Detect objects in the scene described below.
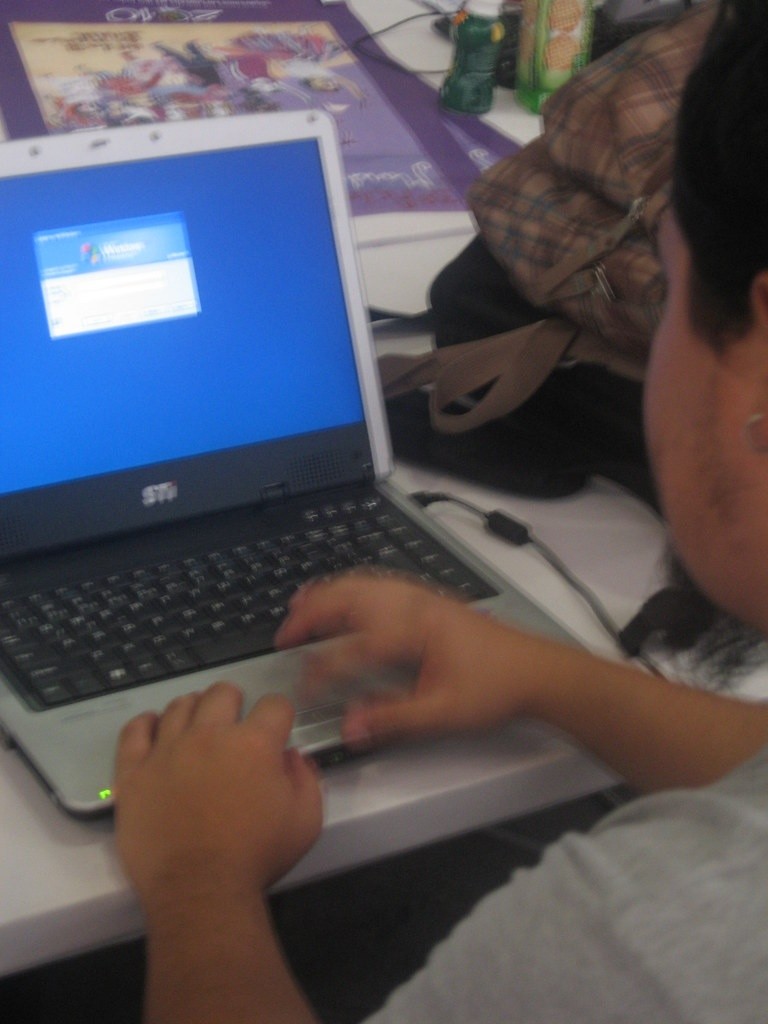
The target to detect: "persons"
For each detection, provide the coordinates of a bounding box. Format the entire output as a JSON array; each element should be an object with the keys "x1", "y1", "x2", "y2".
[
  {"x1": 378, "y1": 0, "x2": 726, "y2": 521},
  {"x1": 105, "y1": 0, "x2": 766, "y2": 1024}
]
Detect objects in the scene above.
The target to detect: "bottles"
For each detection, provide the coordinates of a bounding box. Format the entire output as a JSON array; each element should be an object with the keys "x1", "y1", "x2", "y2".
[
  {"x1": 441, "y1": 1, "x2": 506, "y2": 119},
  {"x1": 515, "y1": 0, "x2": 596, "y2": 114}
]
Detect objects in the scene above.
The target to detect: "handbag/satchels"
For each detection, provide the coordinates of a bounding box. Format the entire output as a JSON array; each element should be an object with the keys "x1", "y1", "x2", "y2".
[{"x1": 366, "y1": 0, "x2": 719, "y2": 519}]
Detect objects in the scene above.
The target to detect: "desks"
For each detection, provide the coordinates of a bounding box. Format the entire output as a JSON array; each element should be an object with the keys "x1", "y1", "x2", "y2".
[{"x1": 2, "y1": 0, "x2": 767, "y2": 982}]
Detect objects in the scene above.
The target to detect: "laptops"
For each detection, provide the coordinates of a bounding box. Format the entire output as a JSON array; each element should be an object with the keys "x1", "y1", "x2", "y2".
[{"x1": 1, "y1": 106, "x2": 594, "y2": 822}]
